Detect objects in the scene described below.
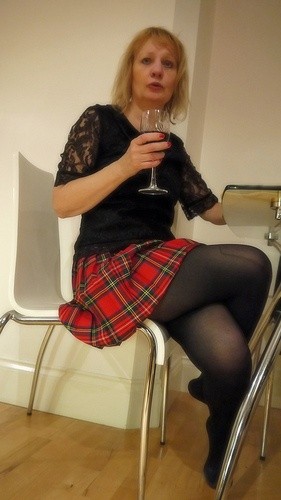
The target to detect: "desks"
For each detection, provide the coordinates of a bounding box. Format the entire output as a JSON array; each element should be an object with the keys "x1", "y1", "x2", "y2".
[{"x1": 212, "y1": 183, "x2": 281, "y2": 500}]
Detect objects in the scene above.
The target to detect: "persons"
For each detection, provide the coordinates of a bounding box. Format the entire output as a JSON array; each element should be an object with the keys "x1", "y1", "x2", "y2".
[{"x1": 51, "y1": 26, "x2": 272, "y2": 490}]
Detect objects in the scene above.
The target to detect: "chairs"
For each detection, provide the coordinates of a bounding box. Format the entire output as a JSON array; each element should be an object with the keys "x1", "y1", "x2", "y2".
[{"x1": 0, "y1": 152, "x2": 173, "y2": 499}]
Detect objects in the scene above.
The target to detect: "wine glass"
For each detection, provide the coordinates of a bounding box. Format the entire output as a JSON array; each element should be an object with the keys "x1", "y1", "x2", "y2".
[{"x1": 137, "y1": 109, "x2": 169, "y2": 195}]
